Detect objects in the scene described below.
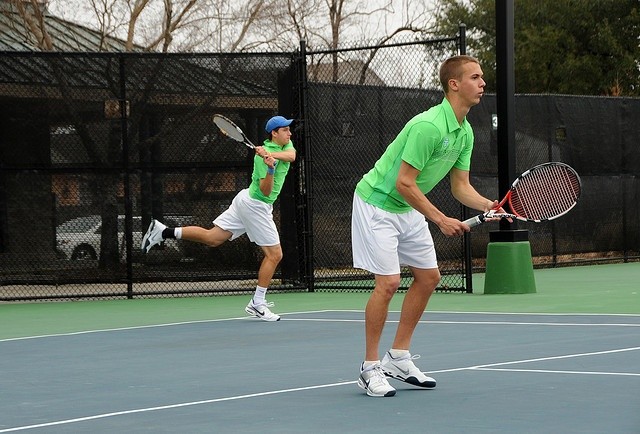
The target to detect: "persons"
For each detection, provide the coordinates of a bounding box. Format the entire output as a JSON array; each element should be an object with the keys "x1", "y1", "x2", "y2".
[
  {"x1": 351, "y1": 56, "x2": 514, "y2": 397},
  {"x1": 141, "y1": 116, "x2": 297, "y2": 322}
]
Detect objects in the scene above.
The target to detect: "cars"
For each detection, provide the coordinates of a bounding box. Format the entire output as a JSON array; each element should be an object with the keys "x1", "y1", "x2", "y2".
[
  {"x1": 158, "y1": 214, "x2": 203, "y2": 227},
  {"x1": 56, "y1": 215, "x2": 194, "y2": 267}
]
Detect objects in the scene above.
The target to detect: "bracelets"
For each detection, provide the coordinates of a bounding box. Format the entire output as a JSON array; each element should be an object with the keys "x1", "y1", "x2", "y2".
[{"x1": 267, "y1": 167, "x2": 275, "y2": 175}]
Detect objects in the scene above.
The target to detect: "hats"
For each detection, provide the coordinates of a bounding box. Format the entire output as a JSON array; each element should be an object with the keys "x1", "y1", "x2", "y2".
[{"x1": 265, "y1": 116, "x2": 295, "y2": 133}]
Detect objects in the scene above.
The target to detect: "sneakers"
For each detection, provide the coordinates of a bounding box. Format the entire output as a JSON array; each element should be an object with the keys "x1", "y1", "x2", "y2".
[
  {"x1": 245, "y1": 298, "x2": 281, "y2": 322},
  {"x1": 381, "y1": 351, "x2": 436, "y2": 389},
  {"x1": 357, "y1": 361, "x2": 396, "y2": 397},
  {"x1": 141, "y1": 220, "x2": 169, "y2": 254}
]
